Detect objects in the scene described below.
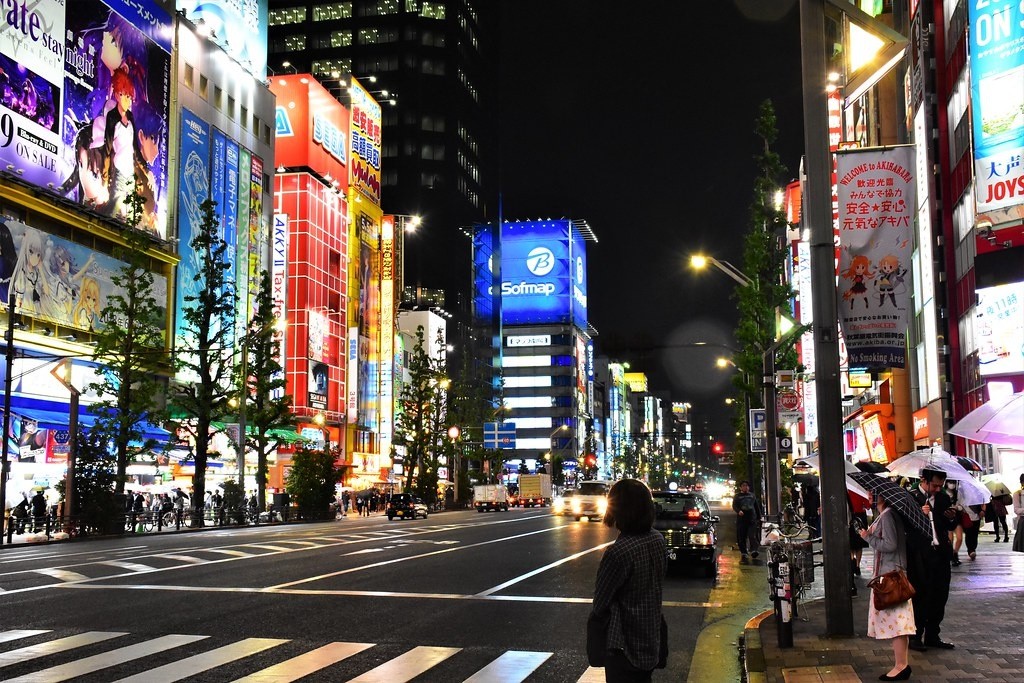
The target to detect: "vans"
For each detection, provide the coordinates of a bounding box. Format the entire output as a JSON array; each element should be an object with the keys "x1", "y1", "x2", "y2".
[{"x1": 574, "y1": 480, "x2": 617, "y2": 522}]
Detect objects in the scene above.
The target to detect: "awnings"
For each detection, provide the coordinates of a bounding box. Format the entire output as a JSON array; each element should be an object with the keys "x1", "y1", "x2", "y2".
[
  {"x1": 0, "y1": 406, "x2": 171, "y2": 442},
  {"x1": 150, "y1": 446, "x2": 225, "y2": 468}
]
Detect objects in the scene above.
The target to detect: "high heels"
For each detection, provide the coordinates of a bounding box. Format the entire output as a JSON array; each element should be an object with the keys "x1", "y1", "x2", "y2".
[{"x1": 878, "y1": 665, "x2": 911, "y2": 681}]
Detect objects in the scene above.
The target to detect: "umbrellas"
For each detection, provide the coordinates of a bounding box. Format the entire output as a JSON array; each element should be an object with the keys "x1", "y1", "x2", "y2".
[
  {"x1": 340, "y1": 486, "x2": 379, "y2": 498},
  {"x1": 803, "y1": 447, "x2": 1012, "y2": 541},
  {"x1": 947, "y1": 393, "x2": 1024, "y2": 446},
  {"x1": 792, "y1": 474, "x2": 818, "y2": 482},
  {"x1": 5, "y1": 484, "x2": 51, "y2": 508},
  {"x1": 124, "y1": 481, "x2": 189, "y2": 499},
  {"x1": 1012, "y1": 474, "x2": 1024, "y2": 553}
]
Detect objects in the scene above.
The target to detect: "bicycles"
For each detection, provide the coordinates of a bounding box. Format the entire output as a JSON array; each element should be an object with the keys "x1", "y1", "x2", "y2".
[
  {"x1": 762, "y1": 522, "x2": 817, "y2": 622},
  {"x1": 162, "y1": 509, "x2": 201, "y2": 528},
  {"x1": 124, "y1": 507, "x2": 155, "y2": 531}
]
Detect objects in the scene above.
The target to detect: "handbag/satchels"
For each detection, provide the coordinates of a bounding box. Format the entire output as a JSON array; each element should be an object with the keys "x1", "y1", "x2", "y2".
[
  {"x1": 946, "y1": 517, "x2": 957, "y2": 531},
  {"x1": 963, "y1": 512, "x2": 973, "y2": 528},
  {"x1": 586, "y1": 608, "x2": 670, "y2": 670},
  {"x1": 867, "y1": 568, "x2": 916, "y2": 610}
]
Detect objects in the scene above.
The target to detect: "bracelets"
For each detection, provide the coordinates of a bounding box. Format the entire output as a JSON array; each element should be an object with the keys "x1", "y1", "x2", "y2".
[{"x1": 981, "y1": 510, "x2": 985, "y2": 512}]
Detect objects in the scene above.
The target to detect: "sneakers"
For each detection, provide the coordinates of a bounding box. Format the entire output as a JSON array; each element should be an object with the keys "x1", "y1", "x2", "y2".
[{"x1": 741, "y1": 555, "x2": 749, "y2": 563}]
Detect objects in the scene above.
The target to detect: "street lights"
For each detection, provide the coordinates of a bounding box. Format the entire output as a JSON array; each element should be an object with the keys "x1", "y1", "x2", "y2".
[
  {"x1": 716, "y1": 355, "x2": 756, "y2": 495},
  {"x1": 550, "y1": 424, "x2": 568, "y2": 480},
  {"x1": 688, "y1": 253, "x2": 781, "y2": 525}
]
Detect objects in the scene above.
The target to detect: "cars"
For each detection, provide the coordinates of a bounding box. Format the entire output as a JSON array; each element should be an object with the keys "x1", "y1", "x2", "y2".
[
  {"x1": 511, "y1": 491, "x2": 518, "y2": 508},
  {"x1": 560, "y1": 489, "x2": 575, "y2": 516},
  {"x1": 386, "y1": 494, "x2": 429, "y2": 521},
  {"x1": 652, "y1": 490, "x2": 720, "y2": 579}
]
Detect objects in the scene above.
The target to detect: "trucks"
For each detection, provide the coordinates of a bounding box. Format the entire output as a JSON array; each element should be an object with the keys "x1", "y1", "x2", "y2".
[
  {"x1": 518, "y1": 473, "x2": 553, "y2": 508},
  {"x1": 473, "y1": 484, "x2": 509, "y2": 513}
]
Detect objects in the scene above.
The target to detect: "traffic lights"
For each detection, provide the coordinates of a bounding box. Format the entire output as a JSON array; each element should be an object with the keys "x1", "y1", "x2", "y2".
[
  {"x1": 588, "y1": 455, "x2": 596, "y2": 466},
  {"x1": 713, "y1": 443, "x2": 722, "y2": 452}
]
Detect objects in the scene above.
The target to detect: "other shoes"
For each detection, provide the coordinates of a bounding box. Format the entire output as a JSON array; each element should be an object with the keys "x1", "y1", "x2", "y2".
[
  {"x1": 969, "y1": 552, "x2": 976, "y2": 561},
  {"x1": 952, "y1": 560, "x2": 961, "y2": 567},
  {"x1": 995, "y1": 537, "x2": 1000, "y2": 542},
  {"x1": 751, "y1": 551, "x2": 759, "y2": 558},
  {"x1": 909, "y1": 640, "x2": 928, "y2": 652},
  {"x1": 1004, "y1": 537, "x2": 1009, "y2": 542},
  {"x1": 924, "y1": 640, "x2": 955, "y2": 649}
]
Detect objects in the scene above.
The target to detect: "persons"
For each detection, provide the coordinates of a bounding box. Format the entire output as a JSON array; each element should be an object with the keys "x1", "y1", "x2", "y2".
[
  {"x1": 991, "y1": 495, "x2": 1009, "y2": 543},
  {"x1": 250, "y1": 489, "x2": 258, "y2": 506},
  {"x1": 944, "y1": 479, "x2": 963, "y2": 566},
  {"x1": 908, "y1": 469, "x2": 955, "y2": 652},
  {"x1": 733, "y1": 480, "x2": 762, "y2": 563},
  {"x1": 802, "y1": 480, "x2": 822, "y2": 543},
  {"x1": 12, "y1": 491, "x2": 47, "y2": 535},
  {"x1": 858, "y1": 496, "x2": 917, "y2": 681},
  {"x1": 342, "y1": 491, "x2": 380, "y2": 516},
  {"x1": 586, "y1": 479, "x2": 668, "y2": 683},
  {"x1": 848, "y1": 490, "x2": 873, "y2": 575},
  {"x1": 204, "y1": 489, "x2": 222, "y2": 523},
  {"x1": 962, "y1": 504, "x2": 986, "y2": 559},
  {"x1": 125, "y1": 490, "x2": 184, "y2": 527}
]
